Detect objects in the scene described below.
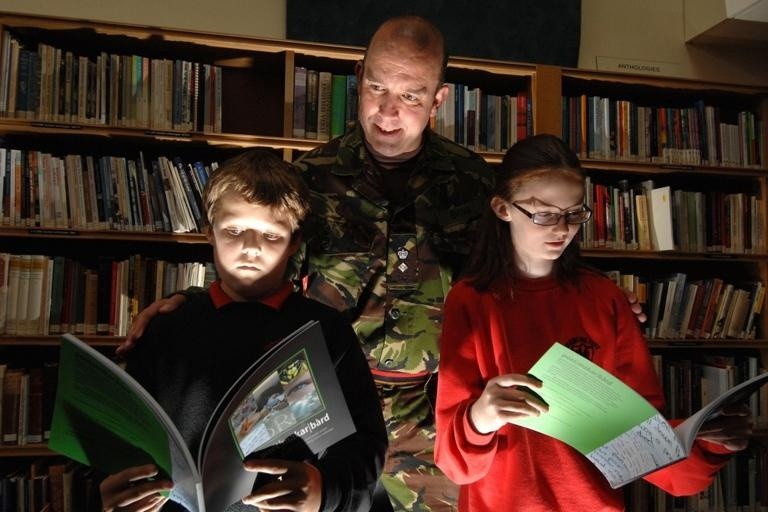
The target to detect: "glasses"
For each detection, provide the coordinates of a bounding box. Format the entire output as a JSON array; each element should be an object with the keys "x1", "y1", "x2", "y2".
[{"x1": 511, "y1": 202, "x2": 592, "y2": 226}]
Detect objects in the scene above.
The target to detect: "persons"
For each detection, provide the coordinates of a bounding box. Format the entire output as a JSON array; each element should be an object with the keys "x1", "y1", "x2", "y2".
[
  {"x1": 68, "y1": 146, "x2": 389, "y2": 512},
  {"x1": 116, "y1": 15, "x2": 647, "y2": 512},
  {"x1": 433, "y1": 136, "x2": 752, "y2": 512}
]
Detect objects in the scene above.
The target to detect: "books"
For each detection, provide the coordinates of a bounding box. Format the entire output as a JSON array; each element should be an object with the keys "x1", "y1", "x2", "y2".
[
  {"x1": 623, "y1": 442, "x2": 768, "y2": 512},
  {"x1": 294, "y1": 66, "x2": 358, "y2": 143},
  {"x1": 48, "y1": 320, "x2": 356, "y2": 512},
  {"x1": 0, "y1": 146, "x2": 219, "y2": 236},
  {"x1": 0, "y1": 31, "x2": 223, "y2": 135},
  {"x1": 429, "y1": 82, "x2": 533, "y2": 152},
  {"x1": 562, "y1": 94, "x2": 765, "y2": 170},
  {"x1": 1, "y1": 364, "x2": 101, "y2": 512},
  {"x1": 579, "y1": 178, "x2": 767, "y2": 256},
  {"x1": 606, "y1": 270, "x2": 766, "y2": 340},
  {"x1": 504, "y1": 342, "x2": 768, "y2": 488},
  {"x1": 0, "y1": 252, "x2": 220, "y2": 339},
  {"x1": 647, "y1": 353, "x2": 768, "y2": 426}
]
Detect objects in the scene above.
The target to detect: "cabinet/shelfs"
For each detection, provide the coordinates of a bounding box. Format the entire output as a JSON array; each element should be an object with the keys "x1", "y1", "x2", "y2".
[{"x1": 0, "y1": 10, "x2": 768, "y2": 512}]
